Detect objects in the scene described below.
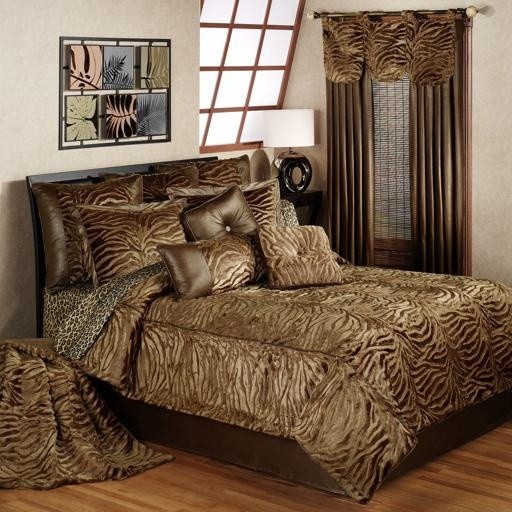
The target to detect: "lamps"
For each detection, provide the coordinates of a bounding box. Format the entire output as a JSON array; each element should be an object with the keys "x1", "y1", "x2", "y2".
[{"x1": 257, "y1": 107, "x2": 316, "y2": 196}]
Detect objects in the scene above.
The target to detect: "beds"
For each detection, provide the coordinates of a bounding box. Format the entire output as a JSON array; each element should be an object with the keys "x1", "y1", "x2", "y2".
[{"x1": 27, "y1": 156, "x2": 512, "y2": 504}]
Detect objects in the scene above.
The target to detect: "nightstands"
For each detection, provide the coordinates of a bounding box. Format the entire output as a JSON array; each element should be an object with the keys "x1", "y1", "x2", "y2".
[{"x1": 280, "y1": 190, "x2": 323, "y2": 225}]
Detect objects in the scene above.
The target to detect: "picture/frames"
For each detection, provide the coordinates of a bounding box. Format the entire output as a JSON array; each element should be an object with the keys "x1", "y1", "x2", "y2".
[{"x1": 56, "y1": 35, "x2": 172, "y2": 152}]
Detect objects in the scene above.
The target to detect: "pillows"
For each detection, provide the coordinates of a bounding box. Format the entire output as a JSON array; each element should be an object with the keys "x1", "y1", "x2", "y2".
[{"x1": 31, "y1": 155, "x2": 343, "y2": 300}]
